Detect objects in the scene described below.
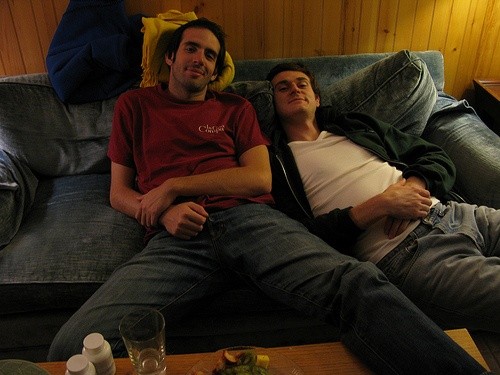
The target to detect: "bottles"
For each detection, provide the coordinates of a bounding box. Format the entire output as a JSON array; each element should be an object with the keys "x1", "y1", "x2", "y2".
[
  {"x1": 82, "y1": 333, "x2": 116, "y2": 375},
  {"x1": 64, "y1": 354, "x2": 96, "y2": 375}
]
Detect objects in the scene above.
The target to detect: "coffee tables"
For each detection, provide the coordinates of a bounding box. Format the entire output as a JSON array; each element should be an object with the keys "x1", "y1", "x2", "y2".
[{"x1": 37, "y1": 328, "x2": 490, "y2": 375}]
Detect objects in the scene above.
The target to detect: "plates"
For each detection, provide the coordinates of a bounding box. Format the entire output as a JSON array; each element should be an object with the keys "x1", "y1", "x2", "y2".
[{"x1": 185, "y1": 346, "x2": 307, "y2": 375}]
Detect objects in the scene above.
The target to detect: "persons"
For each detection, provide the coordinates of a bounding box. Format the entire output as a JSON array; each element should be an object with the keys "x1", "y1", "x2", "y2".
[
  {"x1": 266, "y1": 62, "x2": 500, "y2": 322},
  {"x1": 46, "y1": 19, "x2": 413, "y2": 375}
]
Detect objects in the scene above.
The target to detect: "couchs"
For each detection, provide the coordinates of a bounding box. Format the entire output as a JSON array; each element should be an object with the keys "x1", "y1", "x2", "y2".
[{"x1": 0, "y1": 51, "x2": 500, "y2": 363}]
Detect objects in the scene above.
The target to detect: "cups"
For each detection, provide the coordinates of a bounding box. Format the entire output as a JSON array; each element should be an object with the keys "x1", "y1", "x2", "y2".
[{"x1": 119, "y1": 308, "x2": 167, "y2": 375}]
